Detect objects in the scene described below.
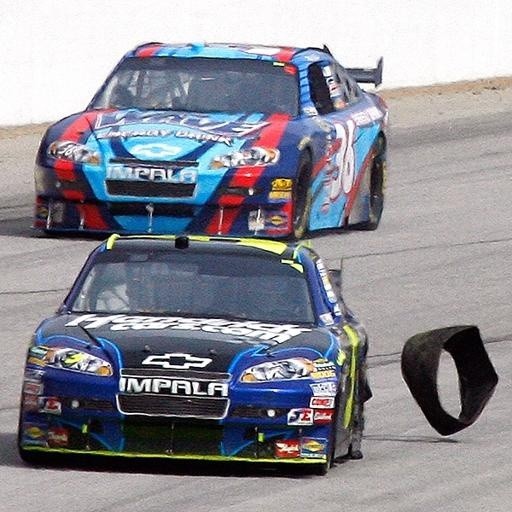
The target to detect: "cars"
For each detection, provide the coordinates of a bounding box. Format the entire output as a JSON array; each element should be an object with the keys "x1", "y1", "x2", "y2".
[
  {"x1": 17, "y1": 234, "x2": 371, "y2": 474},
  {"x1": 29, "y1": 40, "x2": 391, "y2": 239}
]
